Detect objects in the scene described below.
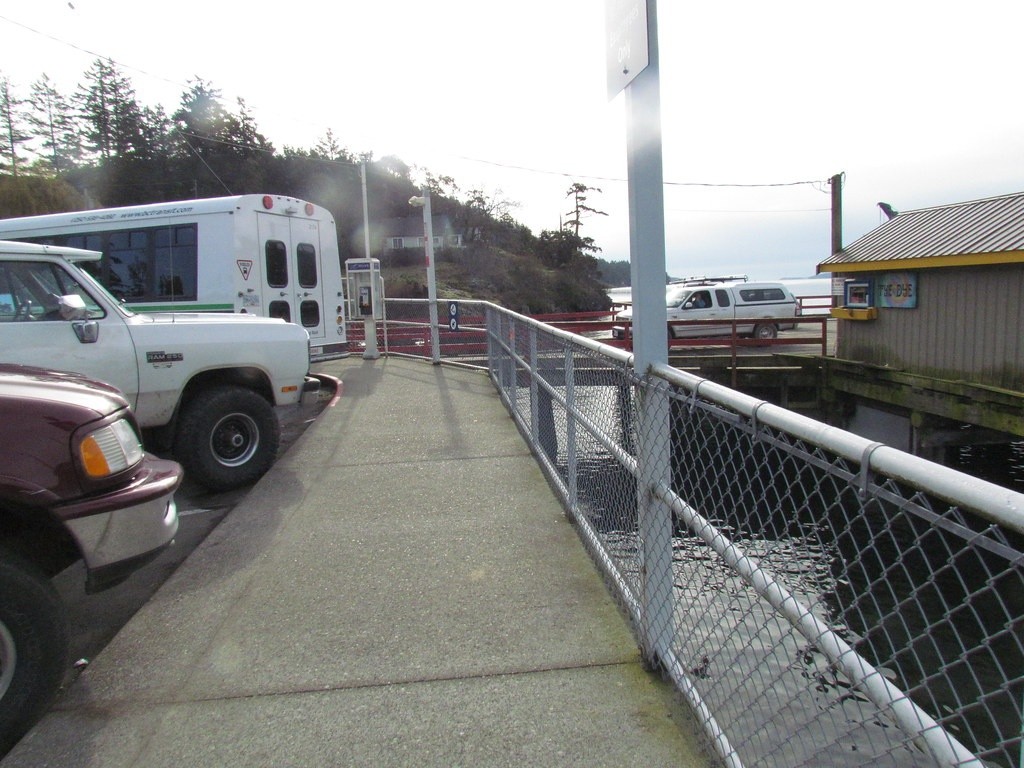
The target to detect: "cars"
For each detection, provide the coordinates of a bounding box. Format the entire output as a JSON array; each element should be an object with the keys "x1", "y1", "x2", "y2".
[{"x1": 0, "y1": 361, "x2": 184, "y2": 730}]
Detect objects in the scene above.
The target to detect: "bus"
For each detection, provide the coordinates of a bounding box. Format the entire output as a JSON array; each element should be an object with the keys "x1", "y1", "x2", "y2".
[{"x1": 0, "y1": 191, "x2": 352, "y2": 364}]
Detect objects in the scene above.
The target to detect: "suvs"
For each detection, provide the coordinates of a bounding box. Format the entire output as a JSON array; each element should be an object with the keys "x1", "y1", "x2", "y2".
[{"x1": 1, "y1": 239, "x2": 323, "y2": 495}]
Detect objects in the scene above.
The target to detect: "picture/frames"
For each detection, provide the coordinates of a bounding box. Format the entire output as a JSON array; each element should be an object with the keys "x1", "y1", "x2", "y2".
[{"x1": 843, "y1": 278, "x2": 874, "y2": 309}]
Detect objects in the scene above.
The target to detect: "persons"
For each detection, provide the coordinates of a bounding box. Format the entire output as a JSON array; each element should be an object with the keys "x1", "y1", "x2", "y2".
[{"x1": 692, "y1": 294, "x2": 706, "y2": 307}]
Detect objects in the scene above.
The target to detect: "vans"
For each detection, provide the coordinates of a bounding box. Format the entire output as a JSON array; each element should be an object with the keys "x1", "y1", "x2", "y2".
[{"x1": 612, "y1": 275, "x2": 803, "y2": 345}]
